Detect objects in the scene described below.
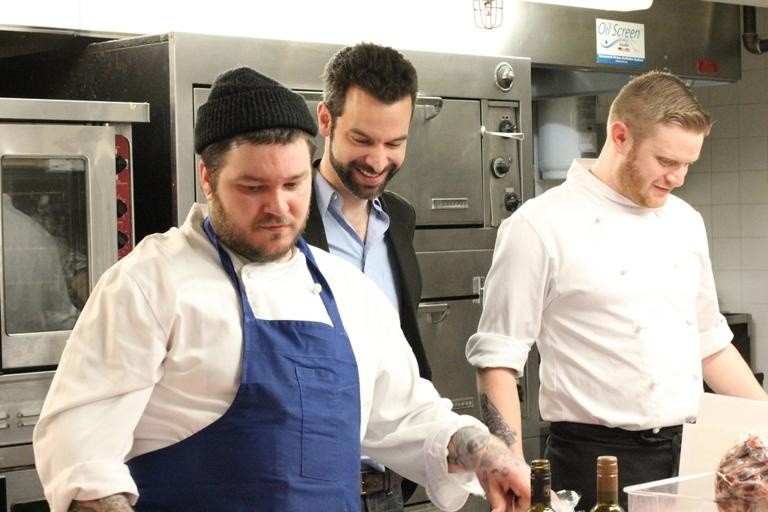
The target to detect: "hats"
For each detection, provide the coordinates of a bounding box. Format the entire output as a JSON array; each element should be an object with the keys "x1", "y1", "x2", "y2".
[{"x1": 195, "y1": 67, "x2": 317, "y2": 153}]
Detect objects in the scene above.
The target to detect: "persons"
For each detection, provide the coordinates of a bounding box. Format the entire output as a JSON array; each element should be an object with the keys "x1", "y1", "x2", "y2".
[
  {"x1": 466, "y1": 67, "x2": 768, "y2": 512},
  {"x1": 3, "y1": 168, "x2": 82, "y2": 334},
  {"x1": 302, "y1": 43, "x2": 434, "y2": 512}
]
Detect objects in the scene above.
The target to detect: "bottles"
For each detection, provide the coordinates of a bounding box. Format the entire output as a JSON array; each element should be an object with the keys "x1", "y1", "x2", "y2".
[
  {"x1": 589, "y1": 454, "x2": 623, "y2": 512},
  {"x1": 524, "y1": 459, "x2": 556, "y2": 512}
]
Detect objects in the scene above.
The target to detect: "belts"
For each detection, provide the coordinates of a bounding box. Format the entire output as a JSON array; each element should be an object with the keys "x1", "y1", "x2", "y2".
[
  {"x1": 551, "y1": 421, "x2": 683, "y2": 443},
  {"x1": 360, "y1": 470, "x2": 404, "y2": 495}
]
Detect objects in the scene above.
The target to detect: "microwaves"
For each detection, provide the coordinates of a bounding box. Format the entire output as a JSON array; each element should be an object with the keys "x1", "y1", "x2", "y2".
[{"x1": 0, "y1": 92, "x2": 150, "y2": 366}]
